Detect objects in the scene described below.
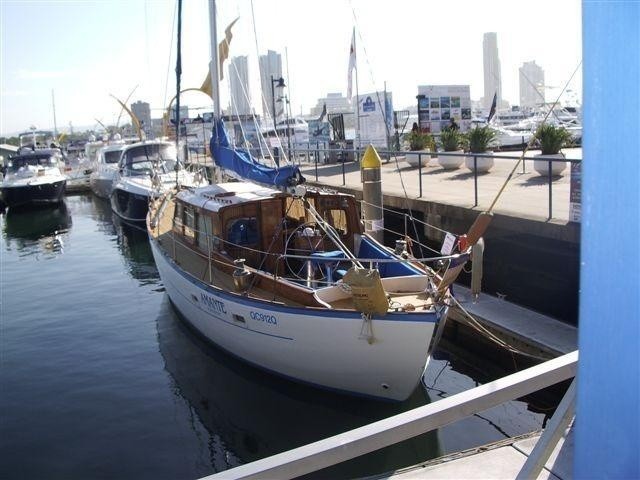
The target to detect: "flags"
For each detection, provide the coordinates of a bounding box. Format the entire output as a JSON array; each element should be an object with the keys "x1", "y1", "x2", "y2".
[
  {"x1": 488, "y1": 92, "x2": 498, "y2": 124},
  {"x1": 346, "y1": 27, "x2": 357, "y2": 101}
]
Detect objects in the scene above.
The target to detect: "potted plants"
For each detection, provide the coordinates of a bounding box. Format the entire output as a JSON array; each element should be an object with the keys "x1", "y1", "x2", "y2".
[
  {"x1": 534, "y1": 124, "x2": 571, "y2": 176},
  {"x1": 438, "y1": 126, "x2": 464, "y2": 170},
  {"x1": 463, "y1": 124, "x2": 501, "y2": 172},
  {"x1": 405, "y1": 130, "x2": 434, "y2": 168}
]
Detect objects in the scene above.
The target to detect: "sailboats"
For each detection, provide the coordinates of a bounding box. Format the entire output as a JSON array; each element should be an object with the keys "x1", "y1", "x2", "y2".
[
  {"x1": 153, "y1": 292, "x2": 445, "y2": 480},
  {"x1": 145, "y1": 1, "x2": 474, "y2": 415}
]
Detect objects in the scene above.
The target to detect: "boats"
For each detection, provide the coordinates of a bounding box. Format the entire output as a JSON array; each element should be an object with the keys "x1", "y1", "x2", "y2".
[
  {"x1": 112, "y1": 215, "x2": 161, "y2": 288},
  {"x1": 110, "y1": 138, "x2": 208, "y2": 234},
  {"x1": 4, "y1": 201, "x2": 72, "y2": 258},
  {"x1": 0, "y1": 87, "x2": 145, "y2": 210},
  {"x1": 472, "y1": 104, "x2": 581, "y2": 153},
  {"x1": 261, "y1": 117, "x2": 329, "y2": 150}
]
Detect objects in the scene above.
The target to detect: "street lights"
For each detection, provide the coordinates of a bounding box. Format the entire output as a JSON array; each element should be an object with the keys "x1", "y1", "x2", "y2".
[
  {"x1": 275, "y1": 93, "x2": 291, "y2": 164},
  {"x1": 269, "y1": 73, "x2": 286, "y2": 168}
]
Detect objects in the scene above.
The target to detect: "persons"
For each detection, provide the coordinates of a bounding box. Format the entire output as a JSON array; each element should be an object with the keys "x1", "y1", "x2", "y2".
[
  {"x1": 113, "y1": 131, "x2": 122, "y2": 140},
  {"x1": 44, "y1": 230, "x2": 64, "y2": 256},
  {"x1": 412, "y1": 122, "x2": 419, "y2": 137},
  {"x1": 87, "y1": 132, "x2": 96, "y2": 142},
  {"x1": 448, "y1": 117, "x2": 458, "y2": 131}
]
